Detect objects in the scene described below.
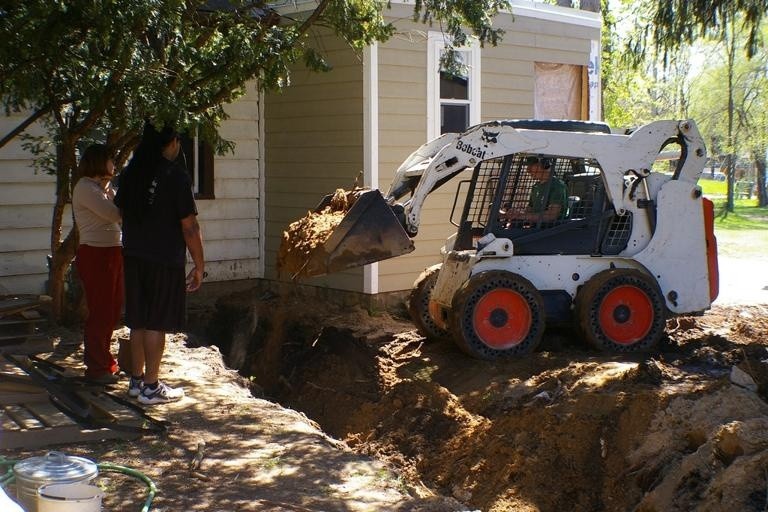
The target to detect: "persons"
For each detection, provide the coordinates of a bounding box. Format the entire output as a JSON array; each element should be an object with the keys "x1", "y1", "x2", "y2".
[
  {"x1": 113, "y1": 124, "x2": 206, "y2": 405},
  {"x1": 71, "y1": 143, "x2": 126, "y2": 383},
  {"x1": 499, "y1": 158, "x2": 576, "y2": 229}
]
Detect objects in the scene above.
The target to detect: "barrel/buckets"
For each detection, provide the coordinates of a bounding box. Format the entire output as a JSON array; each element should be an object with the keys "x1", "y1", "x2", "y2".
[
  {"x1": 12, "y1": 449, "x2": 99, "y2": 511},
  {"x1": 35, "y1": 483, "x2": 104, "y2": 511}
]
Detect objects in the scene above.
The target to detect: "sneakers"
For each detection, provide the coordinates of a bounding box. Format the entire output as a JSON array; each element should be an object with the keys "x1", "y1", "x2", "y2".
[
  {"x1": 138, "y1": 383, "x2": 183, "y2": 404},
  {"x1": 129, "y1": 379, "x2": 143, "y2": 395},
  {"x1": 87, "y1": 370, "x2": 127, "y2": 383}
]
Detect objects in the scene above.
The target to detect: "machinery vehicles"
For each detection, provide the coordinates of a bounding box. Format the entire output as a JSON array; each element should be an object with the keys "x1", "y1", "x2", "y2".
[{"x1": 281, "y1": 117, "x2": 722, "y2": 363}]
